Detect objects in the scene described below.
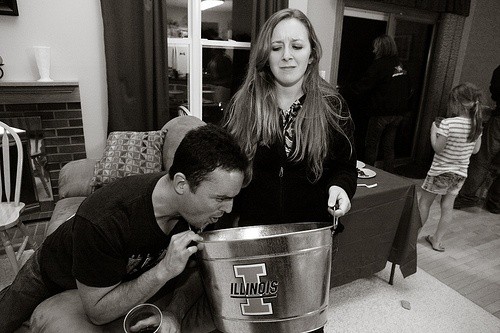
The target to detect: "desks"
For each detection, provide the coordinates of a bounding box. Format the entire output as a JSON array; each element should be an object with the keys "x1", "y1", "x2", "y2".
[{"x1": 332, "y1": 160, "x2": 423, "y2": 289}]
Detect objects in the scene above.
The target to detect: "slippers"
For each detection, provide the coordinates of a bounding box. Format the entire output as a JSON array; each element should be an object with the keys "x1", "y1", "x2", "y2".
[{"x1": 425, "y1": 235, "x2": 445, "y2": 251}]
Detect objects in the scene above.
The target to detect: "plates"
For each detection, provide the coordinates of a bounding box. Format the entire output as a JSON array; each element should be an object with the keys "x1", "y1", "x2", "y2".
[
  {"x1": 357, "y1": 169, "x2": 376, "y2": 178},
  {"x1": 357, "y1": 160, "x2": 365, "y2": 168}
]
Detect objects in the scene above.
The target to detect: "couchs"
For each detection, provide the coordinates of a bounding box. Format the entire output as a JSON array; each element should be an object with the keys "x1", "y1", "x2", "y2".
[{"x1": 29, "y1": 116, "x2": 207, "y2": 333}]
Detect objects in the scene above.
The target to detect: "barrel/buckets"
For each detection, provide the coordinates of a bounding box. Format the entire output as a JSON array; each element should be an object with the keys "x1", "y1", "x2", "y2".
[{"x1": 189, "y1": 206, "x2": 338, "y2": 333}]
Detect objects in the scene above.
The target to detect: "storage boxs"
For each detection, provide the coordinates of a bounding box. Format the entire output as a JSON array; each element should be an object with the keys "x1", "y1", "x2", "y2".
[{"x1": 202, "y1": 207, "x2": 338, "y2": 333}]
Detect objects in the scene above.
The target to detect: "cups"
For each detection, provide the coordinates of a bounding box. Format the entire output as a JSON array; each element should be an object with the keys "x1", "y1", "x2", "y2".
[
  {"x1": 123, "y1": 304, "x2": 178, "y2": 333},
  {"x1": 435, "y1": 117, "x2": 445, "y2": 136}
]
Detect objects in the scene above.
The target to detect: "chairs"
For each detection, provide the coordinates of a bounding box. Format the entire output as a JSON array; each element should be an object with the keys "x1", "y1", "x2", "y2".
[{"x1": 0, "y1": 121, "x2": 37, "y2": 277}]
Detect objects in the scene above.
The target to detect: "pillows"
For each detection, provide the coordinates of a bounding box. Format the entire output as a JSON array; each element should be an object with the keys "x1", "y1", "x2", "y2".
[{"x1": 91, "y1": 129, "x2": 168, "y2": 184}]
